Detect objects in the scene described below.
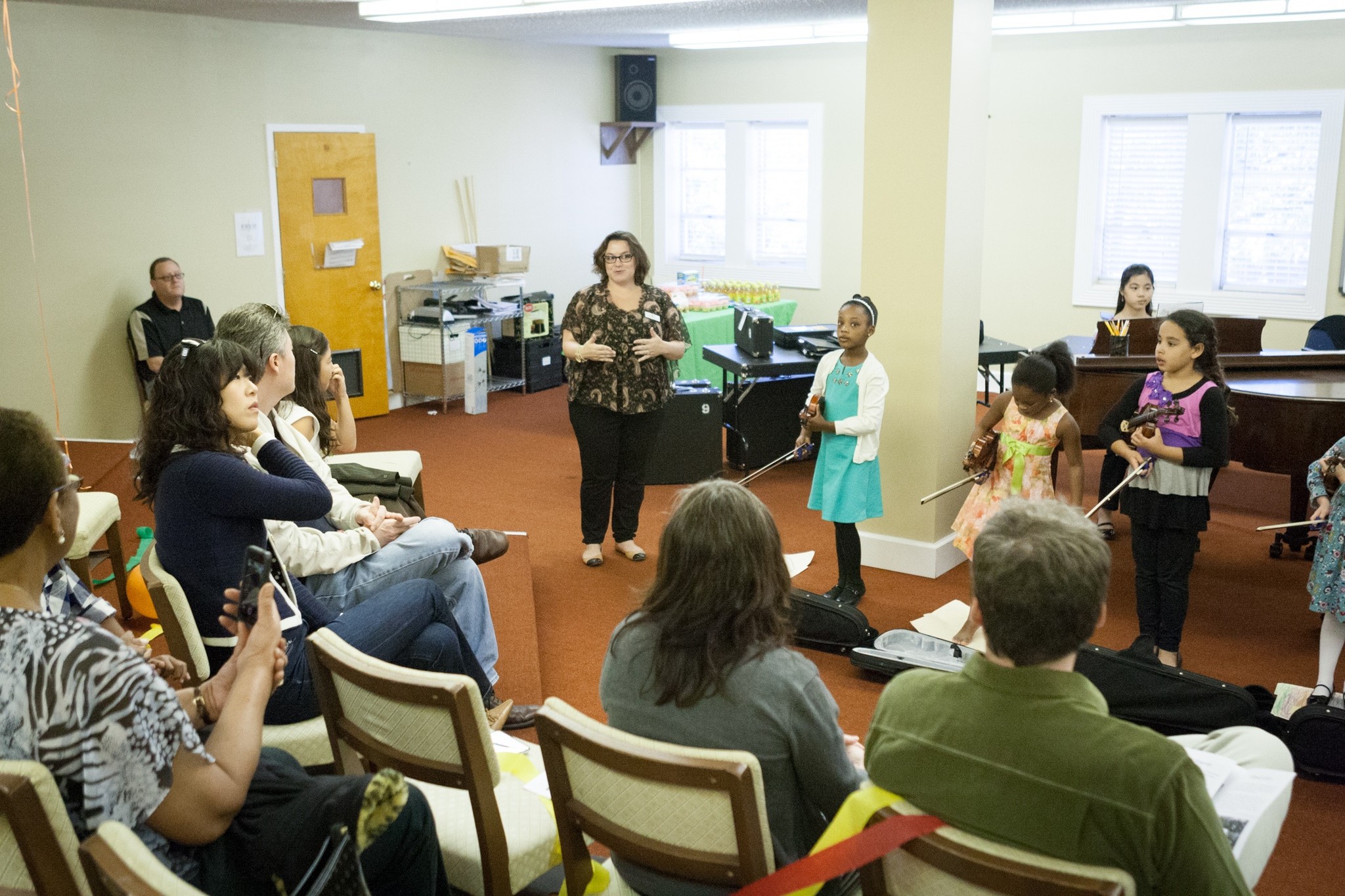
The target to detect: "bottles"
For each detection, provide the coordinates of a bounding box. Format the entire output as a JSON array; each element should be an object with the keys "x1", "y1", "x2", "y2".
[{"x1": 703, "y1": 279, "x2": 782, "y2": 304}]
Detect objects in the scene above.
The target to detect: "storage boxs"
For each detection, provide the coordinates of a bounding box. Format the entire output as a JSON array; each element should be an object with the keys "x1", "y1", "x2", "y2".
[
  {"x1": 734, "y1": 304, "x2": 774, "y2": 357},
  {"x1": 465, "y1": 327, "x2": 488, "y2": 414},
  {"x1": 398, "y1": 325, "x2": 470, "y2": 365},
  {"x1": 773, "y1": 323, "x2": 837, "y2": 351},
  {"x1": 641, "y1": 379, "x2": 724, "y2": 485},
  {"x1": 491, "y1": 337, "x2": 563, "y2": 392},
  {"x1": 501, "y1": 291, "x2": 553, "y2": 339},
  {"x1": 725, "y1": 373, "x2": 819, "y2": 470},
  {"x1": 477, "y1": 245, "x2": 530, "y2": 273}
]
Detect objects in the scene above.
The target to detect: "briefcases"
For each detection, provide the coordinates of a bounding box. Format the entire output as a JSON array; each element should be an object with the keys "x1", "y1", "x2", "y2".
[{"x1": 732, "y1": 305, "x2": 774, "y2": 358}]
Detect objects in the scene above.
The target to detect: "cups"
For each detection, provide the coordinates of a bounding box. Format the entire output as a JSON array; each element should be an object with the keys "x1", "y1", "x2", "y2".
[{"x1": 1109, "y1": 334, "x2": 1129, "y2": 356}]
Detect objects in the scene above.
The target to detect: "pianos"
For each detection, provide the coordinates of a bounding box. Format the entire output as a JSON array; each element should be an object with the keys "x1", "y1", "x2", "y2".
[{"x1": 1026, "y1": 304, "x2": 1345, "y2": 555}]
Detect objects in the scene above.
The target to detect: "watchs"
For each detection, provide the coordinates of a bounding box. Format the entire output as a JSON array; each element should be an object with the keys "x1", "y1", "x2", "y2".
[{"x1": 574, "y1": 346, "x2": 588, "y2": 364}]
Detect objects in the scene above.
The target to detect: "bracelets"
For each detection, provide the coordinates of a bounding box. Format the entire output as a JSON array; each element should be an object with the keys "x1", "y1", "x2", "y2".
[{"x1": 193, "y1": 686, "x2": 214, "y2": 725}]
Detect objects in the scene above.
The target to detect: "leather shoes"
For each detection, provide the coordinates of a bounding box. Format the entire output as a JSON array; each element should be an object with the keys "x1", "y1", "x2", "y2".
[
  {"x1": 1097, "y1": 522, "x2": 1116, "y2": 538},
  {"x1": 459, "y1": 527, "x2": 509, "y2": 564},
  {"x1": 823, "y1": 579, "x2": 866, "y2": 607},
  {"x1": 484, "y1": 696, "x2": 542, "y2": 731}
]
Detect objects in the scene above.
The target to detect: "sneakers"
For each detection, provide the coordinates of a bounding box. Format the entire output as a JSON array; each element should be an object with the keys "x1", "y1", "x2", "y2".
[{"x1": 1306, "y1": 684, "x2": 1335, "y2": 706}]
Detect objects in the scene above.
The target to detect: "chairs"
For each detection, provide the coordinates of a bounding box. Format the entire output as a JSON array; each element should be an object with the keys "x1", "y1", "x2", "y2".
[{"x1": 0, "y1": 451, "x2": 1135, "y2": 896}]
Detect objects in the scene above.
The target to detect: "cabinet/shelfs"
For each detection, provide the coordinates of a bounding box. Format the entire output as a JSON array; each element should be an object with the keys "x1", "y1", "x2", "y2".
[{"x1": 395, "y1": 277, "x2": 527, "y2": 414}]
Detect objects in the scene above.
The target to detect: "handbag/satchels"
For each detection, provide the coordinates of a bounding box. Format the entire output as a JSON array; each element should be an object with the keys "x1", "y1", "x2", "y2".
[
  {"x1": 272, "y1": 824, "x2": 374, "y2": 896},
  {"x1": 784, "y1": 587, "x2": 879, "y2": 657},
  {"x1": 1285, "y1": 704, "x2": 1344, "y2": 786},
  {"x1": 1075, "y1": 643, "x2": 1260, "y2": 740}
]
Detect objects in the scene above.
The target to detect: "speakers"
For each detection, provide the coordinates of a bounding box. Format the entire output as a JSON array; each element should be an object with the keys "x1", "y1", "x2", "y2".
[{"x1": 615, "y1": 54, "x2": 656, "y2": 122}]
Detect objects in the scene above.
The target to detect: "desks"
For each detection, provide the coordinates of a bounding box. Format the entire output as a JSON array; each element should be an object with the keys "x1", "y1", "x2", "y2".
[
  {"x1": 703, "y1": 332, "x2": 842, "y2": 408},
  {"x1": 668, "y1": 296, "x2": 797, "y2": 390},
  {"x1": 976, "y1": 335, "x2": 1029, "y2": 407}
]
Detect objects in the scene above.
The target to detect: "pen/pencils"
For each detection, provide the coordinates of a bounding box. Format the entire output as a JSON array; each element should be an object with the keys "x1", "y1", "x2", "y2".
[{"x1": 1104, "y1": 319, "x2": 1130, "y2": 336}]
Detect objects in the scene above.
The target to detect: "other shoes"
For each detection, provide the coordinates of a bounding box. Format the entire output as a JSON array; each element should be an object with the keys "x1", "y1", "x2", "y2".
[
  {"x1": 486, "y1": 699, "x2": 513, "y2": 732},
  {"x1": 581, "y1": 543, "x2": 604, "y2": 566},
  {"x1": 614, "y1": 540, "x2": 646, "y2": 560}
]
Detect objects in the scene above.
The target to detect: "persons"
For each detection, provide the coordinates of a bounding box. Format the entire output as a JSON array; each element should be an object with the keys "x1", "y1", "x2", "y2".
[
  {"x1": 1304, "y1": 435, "x2": 1345, "y2": 712},
  {"x1": 864, "y1": 500, "x2": 1297, "y2": 896},
  {"x1": 793, "y1": 294, "x2": 888, "y2": 606},
  {"x1": 559, "y1": 231, "x2": 692, "y2": 567},
  {"x1": 1081, "y1": 263, "x2": 1157, "y2": 540},
  {"x1": 599, "y1": 477, "x2": 883, "y2": 896},
  {"x1": 1094, "y1": 308, "x2": 1238, "y2": 670},
  {"x1": 0, "y1": 257, "x2": 548, "y2": 894},
  {"x1": 947, "y1": 338, "x2": 1085, "y2": 647}
]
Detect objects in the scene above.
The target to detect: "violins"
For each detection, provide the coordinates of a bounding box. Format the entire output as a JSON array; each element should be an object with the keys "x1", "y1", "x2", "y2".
[
  {"x1": 1120, "y1": 399, "x2": 1184, "y2": 446},
  {"x1": 1321, "y1": 451, "x2": 1345, "y2": 495},
  {"x1": 798, "y1": 394, "x2": 825, "y2": 428},
  {"x1": 962, "y1": 428, "x2": 1000, "y2": 472}
]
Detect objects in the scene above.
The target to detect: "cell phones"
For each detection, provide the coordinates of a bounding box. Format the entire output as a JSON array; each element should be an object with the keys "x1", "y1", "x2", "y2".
[{"x1": 236, "y1": 545, "x2": 271, "y2": 624}]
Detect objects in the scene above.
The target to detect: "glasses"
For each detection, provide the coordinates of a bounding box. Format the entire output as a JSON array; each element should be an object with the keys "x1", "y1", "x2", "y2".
[
  {"x1": 179, "y1": 337, "x2": 207, "y2": 370},
  {"x1": 48, "y1": 473, "x2": 84, "y2": 497},
  {"x1": 154, "y1": 273, "x2": 185, "y2": 282},
  {"x1": 603, "y1": 253, "x2": 633, "y2": 264}
]
